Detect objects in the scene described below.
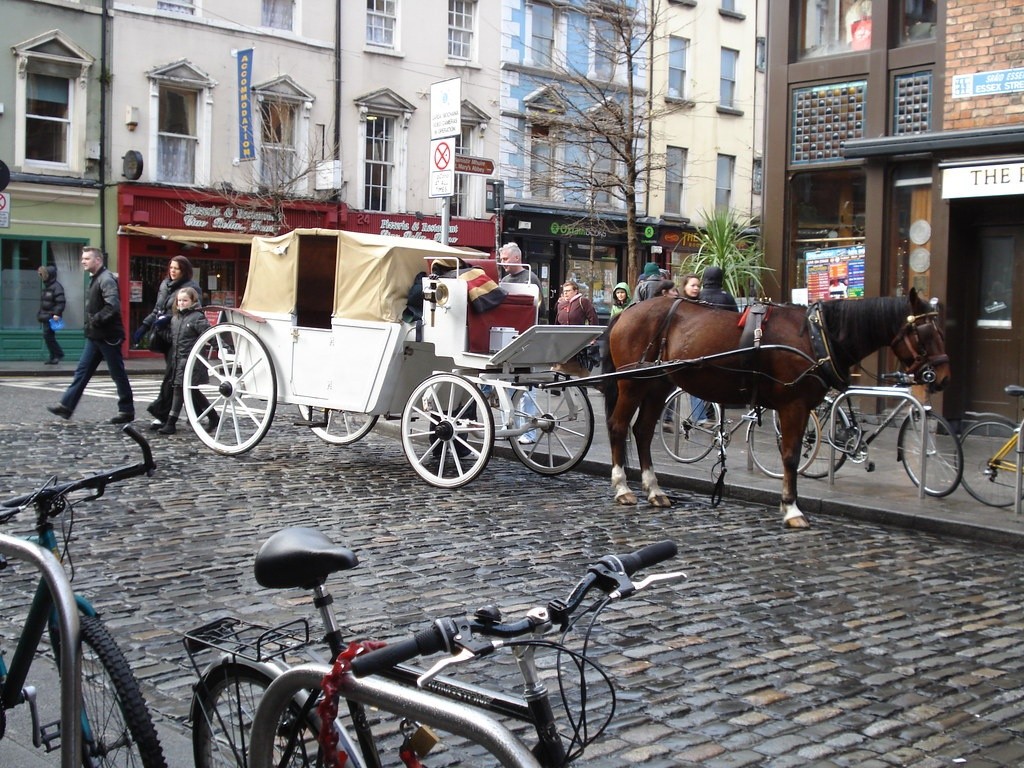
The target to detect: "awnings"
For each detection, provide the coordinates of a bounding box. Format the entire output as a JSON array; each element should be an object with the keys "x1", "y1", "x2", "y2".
[{"x1": 122, "y1": 225, "x2": 279, "y2": 246}]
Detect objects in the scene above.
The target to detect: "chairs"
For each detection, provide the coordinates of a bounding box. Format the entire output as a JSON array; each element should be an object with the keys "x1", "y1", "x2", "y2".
[{"x1": 426, "y1": 258, "x2": 540, "y2": 307}]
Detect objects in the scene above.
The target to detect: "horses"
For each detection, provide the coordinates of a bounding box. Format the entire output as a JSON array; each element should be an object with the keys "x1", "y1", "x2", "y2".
[{"x1": 601, "y1": 289, "x2": 950, "y2": 528}]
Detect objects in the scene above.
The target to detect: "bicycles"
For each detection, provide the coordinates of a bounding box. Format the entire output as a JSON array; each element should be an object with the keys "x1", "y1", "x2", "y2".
[
  {"x1": 660, "y1": 386, "x2": 821, "y2": 479},
  {"x1": 182, "y1": 524, "x2": 678, "y2": 768},
  {"x1": 775, "y1": 371, "x2": 965, "y2": 498},
  {"x1": 0, "y1": 424, "x2": 170, "y2": 768},
  {"x1": 953, "y1": 385, "x2": 1024, "y2": 508}
]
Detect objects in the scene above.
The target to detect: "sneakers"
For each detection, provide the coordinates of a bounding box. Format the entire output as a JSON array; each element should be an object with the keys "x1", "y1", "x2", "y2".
[
  {"x1": 459, "y1": 451, "x2": 480, "y2": 459},
  {"x1": 149, "y1": 418, "x2": 165, "y2": 431},
  {"x1": 433, "y1": 448, "x2": 453, "y2": 457},
  {"x1": 517, "y1": 434, "x2": 534, "y2": 444}
]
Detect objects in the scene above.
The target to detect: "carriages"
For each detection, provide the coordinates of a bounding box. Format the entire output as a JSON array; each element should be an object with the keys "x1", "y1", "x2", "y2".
[{"x1": 184, "y1": 266, "x2": 951, "y2": 528}]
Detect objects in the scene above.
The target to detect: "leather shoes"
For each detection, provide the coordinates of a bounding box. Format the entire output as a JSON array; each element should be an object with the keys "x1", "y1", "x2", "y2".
[
  {"x1": 45, "y1": 354, "x2": 64, "y2": 365},
  {"x1": 110, "y1": 412, "x2": 134, "y2": 423},
  {"x1": 46, "y1": 404, "x2": 73, "y2": 420}
]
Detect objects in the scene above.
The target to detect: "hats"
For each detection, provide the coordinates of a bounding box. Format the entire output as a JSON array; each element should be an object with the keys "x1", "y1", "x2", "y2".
[
  {"x1": 644, "y1": 263, "x2": 660, "y2": 276},
  {"x1": 659, "y1": 269, "x2": 671, "y2": 278},
  {"x1": 638, "y1": 274, "x2": 645, "y2": 283}
]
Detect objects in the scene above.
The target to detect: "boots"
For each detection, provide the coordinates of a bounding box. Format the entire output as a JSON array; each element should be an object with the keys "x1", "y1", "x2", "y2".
[
  {"x1": 203, "y1": 404, "x2": 220, "y2": 432},
  {"x1": 158, "y1": 411, "x2": 179, "y2": 433}
]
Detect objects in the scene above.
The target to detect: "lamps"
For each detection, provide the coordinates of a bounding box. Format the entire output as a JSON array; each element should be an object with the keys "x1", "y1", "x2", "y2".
[
  {"x1": 247, "y1": 184, "x2": 269, "y2": 196},
  {"x1": 211, "y1": 181, "x2": 235, "y2": 194},
  {"x1": 406, "y1": 210, "x2": 426, "y2": 220}
]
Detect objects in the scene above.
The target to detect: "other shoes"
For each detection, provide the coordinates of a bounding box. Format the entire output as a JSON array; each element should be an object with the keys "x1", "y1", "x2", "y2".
[
  {"x1": 698, "y1": 419, "x2": 717, "y2": 431},
  {"x1": 663, "y1": 421, "x2": 682, "y2": 434}
]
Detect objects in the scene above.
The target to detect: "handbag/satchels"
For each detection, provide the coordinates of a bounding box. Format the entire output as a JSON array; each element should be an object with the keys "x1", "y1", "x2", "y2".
[
  {"x1": 50, "y1": 316, "x2": 64, "y2": 332},
  {"x1": 146, "y1": 327, "x2": 173, "y2": 354}
]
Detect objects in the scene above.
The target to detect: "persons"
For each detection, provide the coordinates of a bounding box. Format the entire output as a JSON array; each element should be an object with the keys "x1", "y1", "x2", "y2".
[
  {"x1": 156, "y1": 287, "x2": 235, "y2": 435},
  {"x1": 545, "y1": 281, "x2": 600, "y2": 396},
  {"x1": 609, "y1": 282, "x2": 634, "y2": 325},
  {"x1": 132, "y1": 255, "x2": 202, "y2": 428},
  {"x1": 36, "y1": 265, "x2": 66, "y2": 366},
  {"x1": 46, "y1": 245, "x2": 135, "y2": 424},
  {"x1": 633, "y1": 261, "x2": 680, "y2": 434},
  {"x1": 429, "y1": 242, "x2": 547, "y2": 460},
  {"x1": 677, "y1": 266, "x2": 739, "y2": 426}
]
